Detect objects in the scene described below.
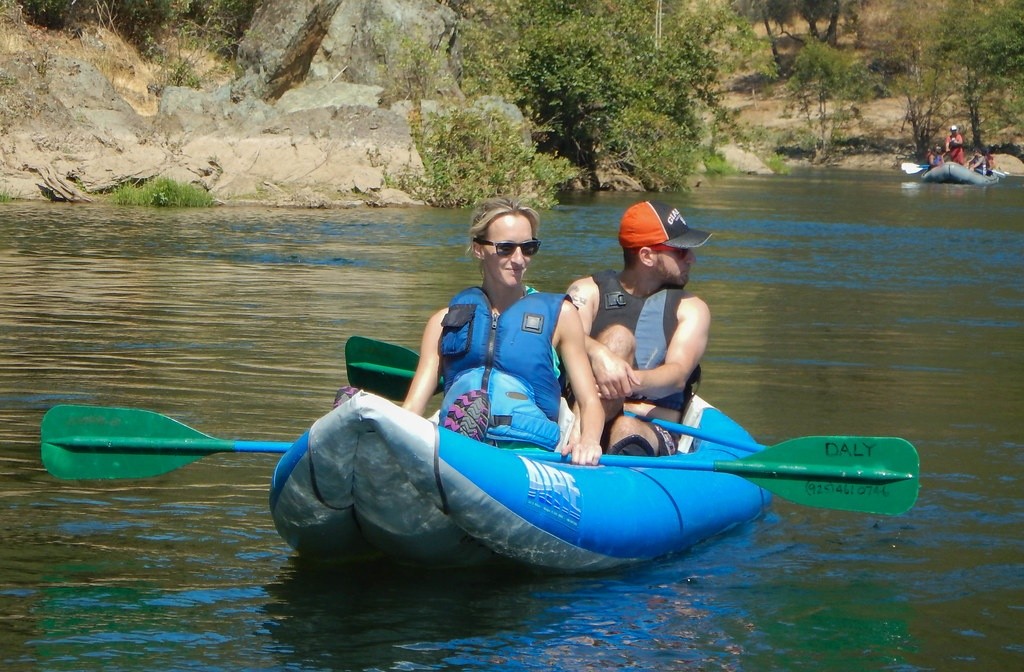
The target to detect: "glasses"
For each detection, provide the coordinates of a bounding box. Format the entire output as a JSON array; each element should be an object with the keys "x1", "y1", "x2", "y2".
[
  {"x1": 473, "y1": 238, "x2": 542, "y2": 256},
  {"x1": 629, "y1": 245, "x2": 689, "y2": 260}
]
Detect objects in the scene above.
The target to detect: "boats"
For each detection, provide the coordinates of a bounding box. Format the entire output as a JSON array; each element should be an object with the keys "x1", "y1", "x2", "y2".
[
  {"x1": 269, "y1": 392, "x2": 773, "y2": 574},
  {"x1": 920, "y1": 160, "x2": 1000, "y2": 186}
]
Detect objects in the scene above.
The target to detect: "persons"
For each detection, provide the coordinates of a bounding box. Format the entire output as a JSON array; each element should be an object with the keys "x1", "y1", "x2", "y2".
[
  {"x1": 945, "y1": 125, "x2": 964, "y2": 165},
  {"x1": 563, "y1": 200, "x2": 712, "y2": 458},
  {"x1": 926, "y1": 144, "x2": 946, "y2": 167},
  {"x1": 333, "y1": 198, "x2": 606, "y2": 468},
  {"x1": 968, "y1": 147, "x2": 995, "y2": 177}
]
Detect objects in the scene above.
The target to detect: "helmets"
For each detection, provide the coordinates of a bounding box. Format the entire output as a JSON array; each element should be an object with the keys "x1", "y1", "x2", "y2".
[{"x1": 951, "y1": 126, "x2": 957, "y2": 131}]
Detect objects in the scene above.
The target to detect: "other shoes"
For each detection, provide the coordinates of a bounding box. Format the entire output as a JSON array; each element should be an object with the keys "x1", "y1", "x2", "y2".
[
  {"x1": 608, "y1": 434, "x2": 655, "y2": 456},
  {"x1": 334, "y1": 386, "x2": 359, "y2": 409},
  {"x1": 443, "y1": 389, "x2": 490, "y2": 441}
]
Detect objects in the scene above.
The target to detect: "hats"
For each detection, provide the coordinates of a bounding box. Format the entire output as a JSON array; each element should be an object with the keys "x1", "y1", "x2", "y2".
[
  {"x1": 619, "y1": 200, "x2": 713, "y2": 251},
  {"x1": 972, "y1": 148, "x2": 980, "y2": 152}
]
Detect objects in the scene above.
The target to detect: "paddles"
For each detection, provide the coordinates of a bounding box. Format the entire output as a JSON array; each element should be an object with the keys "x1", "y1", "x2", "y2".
[
  {"x1": 40, "y1": 402, "x2": 919, "y2": 518},
  {"x1": 901, "y1": 162, "x2": 930, "y2": 174},
  {"x1": 343, "y1": 335, "x2": 769, "y2": 451}
]
